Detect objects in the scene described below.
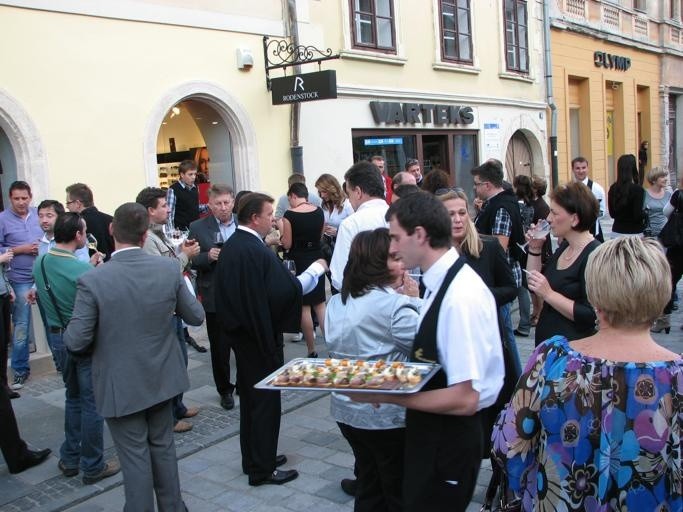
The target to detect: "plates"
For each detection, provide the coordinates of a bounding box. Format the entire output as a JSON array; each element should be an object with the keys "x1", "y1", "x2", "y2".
[{"x1": 253, "y1": 356, "x2": 441, "y2": 395}]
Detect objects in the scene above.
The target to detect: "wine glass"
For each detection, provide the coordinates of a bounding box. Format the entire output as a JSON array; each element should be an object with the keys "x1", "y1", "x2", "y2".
[
  {"x1": 185, "y1": 240, "x2": 195, "y2": 266},
  {"x1": 516, "y1": 219, "x2": 552, "y2": 254},
  {"x1": 213, "y1": 232, "x2": 223, "y2": 249},
  {"x1": 283, "y1": 260, "x2": 297, "y2": 276},
  {"x1": 85, "y1": 233, "x2": 106, "y2": 264}
]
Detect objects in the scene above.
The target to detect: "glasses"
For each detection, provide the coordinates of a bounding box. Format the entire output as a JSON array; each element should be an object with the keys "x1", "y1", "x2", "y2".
[
  {"x1": 474, "y1": 181, "x2": 489, "y2": 186},
  {"x1": 65, "y1": 198, "x2": 79, "y2": 205}
]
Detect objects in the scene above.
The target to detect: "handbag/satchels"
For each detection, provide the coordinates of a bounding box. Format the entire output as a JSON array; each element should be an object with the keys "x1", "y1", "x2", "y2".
[{"x1": 38, "y1": 253, "x2": 96, "y2": 366}]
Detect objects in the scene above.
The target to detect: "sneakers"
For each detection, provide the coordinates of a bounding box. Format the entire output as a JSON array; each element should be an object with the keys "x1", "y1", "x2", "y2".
[
  {"x1": 314, "y1": 331, "x2": 316, "y2": 338},
  {"x1": 83, "y1": 462, "x2": 120, "y2": 483},
  {"x1": 12, "y1": 376, "x2": 24, "y2": 389},
  {"x1": 59, "y1": 459, "x2": 77, "y2": 475},
  {"x1": 292, "y1": 332, "x2": 302, "y2": 341}
]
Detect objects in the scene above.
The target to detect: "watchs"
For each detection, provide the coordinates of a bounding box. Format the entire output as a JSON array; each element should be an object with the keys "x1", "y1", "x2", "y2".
[{"x1": 528, "y1": 251, "x2": 541, "y2": 256}]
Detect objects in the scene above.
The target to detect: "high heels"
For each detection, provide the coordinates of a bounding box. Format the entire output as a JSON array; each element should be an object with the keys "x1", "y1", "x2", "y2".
[{"x1": 651, "y1": 315, "x2": 671, "y2": 334}]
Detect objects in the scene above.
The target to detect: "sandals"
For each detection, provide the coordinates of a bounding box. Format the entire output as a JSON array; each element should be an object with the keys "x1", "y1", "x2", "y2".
[
  {"x1": 529, "y1": 314, "x2": 536, "y2": 322},
  {"x1": 531, "y1": 317, "x2": 539, "y2": 326}
]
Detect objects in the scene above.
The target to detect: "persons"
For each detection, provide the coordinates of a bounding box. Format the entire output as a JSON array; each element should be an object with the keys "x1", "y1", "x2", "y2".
[
  {"x1": 525, "y1": 180, "x2": 602, "y2": 348},
  {"x1": 62, "y1": 203, "x2": 205, "y2": 512},
  {"x1": 419, "y1": 186, "x2": 522, "y2": 413},
  {"x1": 483, "y1": 234, "x2": 682, "y2": 512},
  {"x1": 215, "y1": 193, "x2": 328, "y2": 487},
  {"x1": 422, "y1": 169, "x2": 450, "y2": 194},
  {"x1": 0, "y1": 247, "x2": 21, "y2": 400},
  {"x1": 189, "y1": 184, "x2": 240, "y2": 410},
  {"x1": 390, "y1": 184, "x2": 422, "y2": 203},
  {"x1": 281, "y1": 183, "x2": 327, "y2": 357},
  {"x1": 608, "y1": 154, "x2": 648, "y2": 238},
  {"x1": 472, "y1": 163, "x2": 522, "y2": 407},
  {"x1": 329, "y1": 161, "x2": 389, "y2": 290},
  {"x1": 489, "y1": 159, "x2": 513, "y2": 193},
  {"x1": 66, "y1": 183, "x2": 115, "y2": 265},
  {"x1": 644, "y1": 166, "x2": 674, "y2": 236},
  {"x1": 529, "y1": 176, "x2": 550, "y2": 325},
  {"x1": 32, "y1": 211, "x2": 120, "y2": 485},
  {"x1": 24, "y1": 201, "x2": 89, "y2": 304},
  {"x1": 509, "y1": 175, "x2": 534, "y2": 337},
  {"x1": 406, "y1": 158, "x2": 423, "y2": 186},
  {"x1": 639, "y1": 141, "x2": 648, "y2": 185},
  {"x1": 164, "y1": 160, "x2": 209, "y2": 230},
  {"x1": 0, "y1": 180, "x2": 45, "y2": 389},
  {"x1": 196, "y1": 171, "x2": 211, "y2": 218},
  {"x1": 571, "y1": 157, "x2": 605, "y2": 243},
  {"x1": 325, "y1": 228, "x2": 424, "y2": 511},
  {"x1": 138, "y1": 186, "x2": 200, "y2": 431},
  {"x1": 393, "y1": 173, "x2": 416, "y2": 185},
  {"x1": 1, "y1": 378, "x2": 51, "y2": 474},
  {"x1": 371, "y1": 156, "x2": 392, "y2": 204},
  {"x1": 232, "y1": 189, "x2": 279, "y2": 247},
  {"x1": 315, "y1": 173, "x2": 355, "y2": 255},
  {"x1": 334, "y1": 191, "x2": 505, "y2": 512},
  {"x1": 273, "y1": 174, "x2": 307, "y2": 229},
  {"x1": 651, "y1": 176, "x2": 682, "y2": 334}
]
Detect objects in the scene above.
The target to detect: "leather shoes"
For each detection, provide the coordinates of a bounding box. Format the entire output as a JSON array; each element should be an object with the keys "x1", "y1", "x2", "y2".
[
  {"x1": 221, "y1": 393, "x2": 234, "y2": 408},
  {"x1": 308, "y1": 351, "x2": 318, "y2": 358},
  {"x1": 9, "y1": 447, "x2": 51, "y2": 474},
  {"x1": 248, "y1": 467, "x2": 298, "y2": 486},
  {"x1": 8, "y1": 388, "x2": 19, "y2": 397},
  {"x1": 275, "y1": 454, "x2": 287, "y2": 466},
  {"x1": 184, "y1": 407, "x2": 198, "y2": 418},
  {"x1": 341, "y1": 476, "x2": 357, "y2": 494},
  {"x1": 173, "y1": 420, "x2": 192, "y2": 431},
  {"x1": 513, "y1": 329, "x2": 528, "y2": 337}
]
